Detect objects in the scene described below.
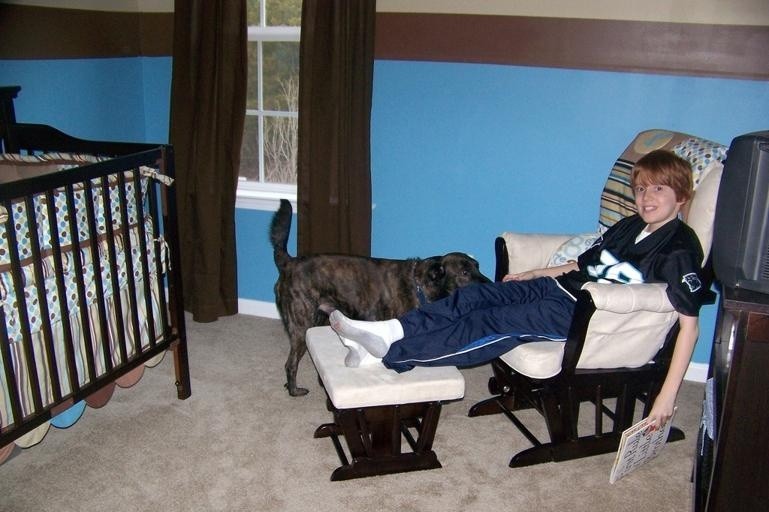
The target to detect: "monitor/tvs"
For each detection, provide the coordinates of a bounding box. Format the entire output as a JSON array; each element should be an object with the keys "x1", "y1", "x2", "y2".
[{"x1": 707, "y1": 131, "x2": 769, "y2": 303}]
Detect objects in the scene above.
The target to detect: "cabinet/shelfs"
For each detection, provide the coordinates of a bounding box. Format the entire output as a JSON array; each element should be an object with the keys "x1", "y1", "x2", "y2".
[{"x1": 688, "y1": 279, "x2": 769, "y2": 512}]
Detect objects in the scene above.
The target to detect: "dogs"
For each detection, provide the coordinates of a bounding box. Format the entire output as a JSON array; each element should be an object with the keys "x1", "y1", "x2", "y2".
[{"x1": 270, "y1": 200, "x2": 495, "y2": 398}]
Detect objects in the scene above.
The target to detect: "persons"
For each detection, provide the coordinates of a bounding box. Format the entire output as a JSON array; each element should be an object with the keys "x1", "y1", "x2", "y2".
[{"x1": 327, "y1": 148, "x2": 705, "y2": 430}]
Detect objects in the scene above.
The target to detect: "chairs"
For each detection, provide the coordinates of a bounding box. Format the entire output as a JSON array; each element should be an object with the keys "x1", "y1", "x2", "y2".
[{"x1": 463, "y1": 128, "x2": 728, "y2": 470}]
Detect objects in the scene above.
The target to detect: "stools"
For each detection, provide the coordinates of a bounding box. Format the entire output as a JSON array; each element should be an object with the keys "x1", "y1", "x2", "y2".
[{"x1": 304, "y1": 323, "x2": 466, "y2": 481}]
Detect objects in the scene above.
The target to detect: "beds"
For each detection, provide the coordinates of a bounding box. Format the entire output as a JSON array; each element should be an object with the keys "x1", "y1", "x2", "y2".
[{"x1": 0, "y1": 118, "x2": 191, "y2": 452}]
